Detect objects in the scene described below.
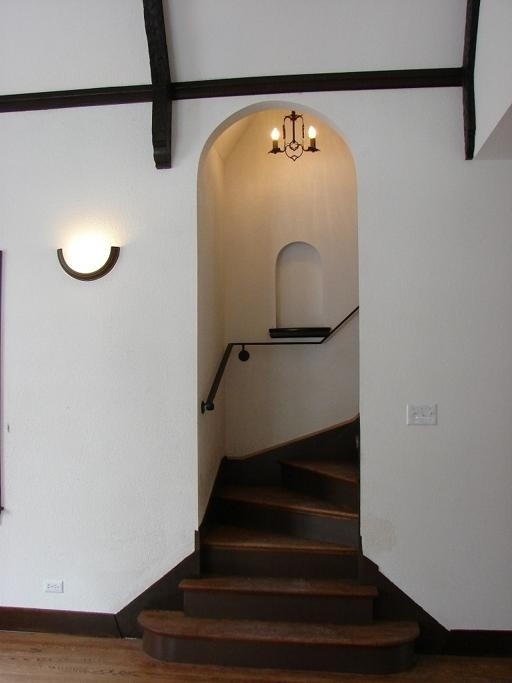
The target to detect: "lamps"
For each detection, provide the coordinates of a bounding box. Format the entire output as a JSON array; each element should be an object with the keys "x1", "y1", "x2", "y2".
[
  {"x1": 266, "y1": 109, "x2": 321, "y2": 162},
  {"x1": 55, "y1": 218, "x2": 123, "y2": 282}
]
